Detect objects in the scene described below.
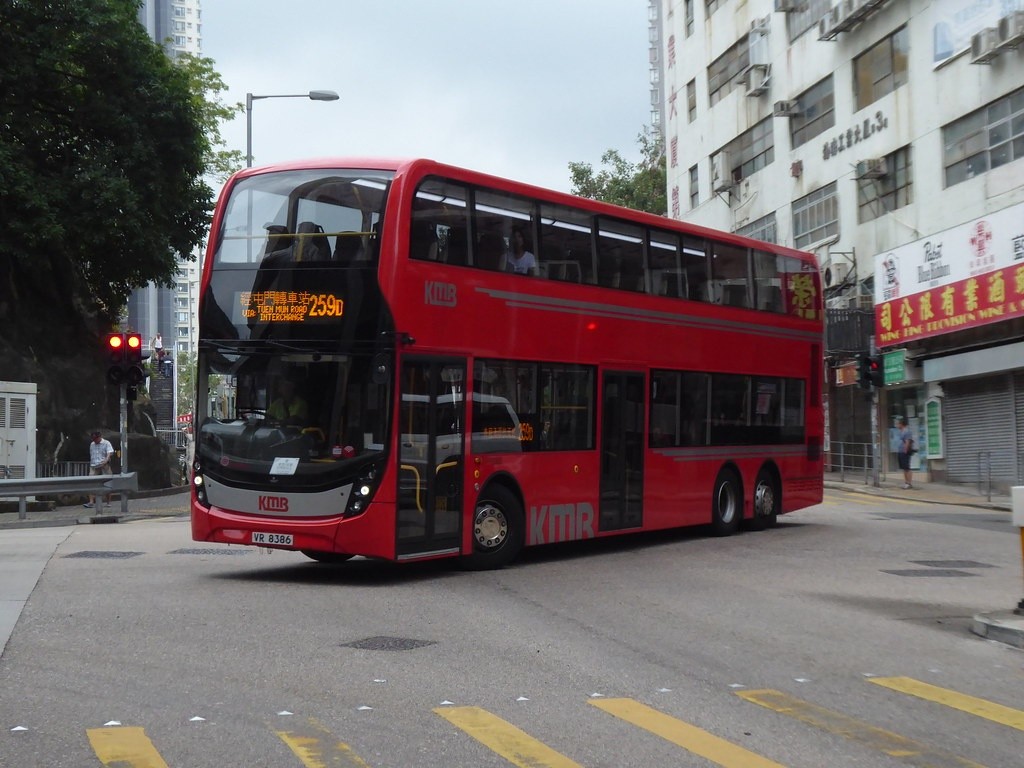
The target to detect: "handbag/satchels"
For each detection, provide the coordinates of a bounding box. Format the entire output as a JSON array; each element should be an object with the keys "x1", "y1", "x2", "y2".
[{"x1": 910, "y1": 443, "x2": 919, "y2": 452}]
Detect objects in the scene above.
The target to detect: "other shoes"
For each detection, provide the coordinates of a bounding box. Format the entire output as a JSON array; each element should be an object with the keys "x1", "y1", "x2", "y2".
[
  {"x1": 83, "y1": 503, "x2": 94, "y2": 508},
  {"x1": 105, "y1": 504, "x2": 110, "y2": 507},
  {"x1": 902, "y1": 483, "x2": 912, "y2": 488}
]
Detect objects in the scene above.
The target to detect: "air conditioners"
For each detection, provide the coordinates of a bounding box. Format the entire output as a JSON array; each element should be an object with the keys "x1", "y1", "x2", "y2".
[
  {"x1": 825, "y1": 296, "x2": 849, "y2": 322},
  {"x1": 750, "y1": 18, "x2": 766, "y2": 34},
  {"x1": 856, "y1": 158, "x2": 887, "y2": 179},
  {"x1": 970, "y1": 27, "x2": 999, "y2": 62},
  {"x1": 745, "y1": 69, "x2": 765, "y2": 97},
  {"x1": 773, "y1": 100, "x2": 799, "y2": 117},
  {"x1": 819, "y1": 12, "x2": 836, "y2": 38},
  {"x1": 996, "y1": 10, "x2": 1024, "y2": 47},
  {"x1": 775, "y1": 0, "x2": 795, "y2": 12},
  {"x1": 832, "y1": 1, "x2": 853, "y2": 30},
  {"x1": 822, "y1": 263, "x2": 847, "y2": 289},
  {"x1": 844, "y1": 0, "x2": 872, "y2": 18}
]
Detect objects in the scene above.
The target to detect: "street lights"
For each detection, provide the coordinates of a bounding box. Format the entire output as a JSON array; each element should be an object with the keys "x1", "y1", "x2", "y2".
[{"x1": 245, "y1": 90, "x2": 341, "y2": 264}]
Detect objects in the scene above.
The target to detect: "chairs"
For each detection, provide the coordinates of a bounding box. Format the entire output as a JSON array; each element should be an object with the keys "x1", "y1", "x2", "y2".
[
  {"x1": 722, "y1": 285, "x2": 746, "y2": 309},
  {"x1": 597, "y1": 270, "x2": 621, "y2": 288},
  {"x1": 311, "y1": 225, "x2": 331, "y2": 260},
  {"x1": 332, "y1": 231, "x2": 364, "y2": 260},
  {"x1": 413, "y1": 221, "x2": 439, "y2": 261},
  {"x1": 447, "y1": 227, "x2": 478, "y2": 265},
  {"x1": 480, "y1": 234, "x2": 593, "y2": 283},
  {"x1": 621, "y1": 274, "x2": 645, "y2": 292},
  {"x1": 759, "y1": 286, "x2": 783, "y2": 312},
  {"x1": 654, "y1": 271, "x2": 687, "y2": 299}
]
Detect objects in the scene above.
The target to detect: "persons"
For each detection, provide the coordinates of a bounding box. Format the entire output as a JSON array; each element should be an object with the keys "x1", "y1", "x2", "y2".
[
  {"x1": 258, "y1": 216, "x2": 319, "y2": 262},
  {"x1": 541, "y1": 230, "x2": 573, "y2": 273},
  {"x1": 548, "y1": 372, "x2": 589, "y2": 425},
  {"x1": 267, "y1": 379, "x2": 310, "y2": 429},
  {"x1": 498, "y1": 227, "x2": 535, "y2": 277},
  {"x1": 894, "y1": 415, "x2": 914, "y2": 490},
  {"x1": 340, "y1": 381, "x2": 385, "y2": 444},
  {"x1": 82, "y1": 431, "x2": 116, "y2": 509},
  {"x1": 598, "y1": 239, "x2": 638, "y2": 292}
]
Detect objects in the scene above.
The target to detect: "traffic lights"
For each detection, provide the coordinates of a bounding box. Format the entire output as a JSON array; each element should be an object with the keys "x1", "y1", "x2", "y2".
[
  {"x1": 105, "y1": 333, "x2": 124, "y2": 385},
  {"x1": 123, "y1": 331, "x2": 144, "y2": 386},
  {"x1": 140, "y1": 349, "x2": 153, "y2": 383},
  {"x1": 853, "y1": 354, "x2": 871, "y2": 390},
  {"x1": 867, "y1": 353, "x2": 886, "y2": 387},
  {"x1": 823, "y1": 356, "x2": 834, "y2": 387}
]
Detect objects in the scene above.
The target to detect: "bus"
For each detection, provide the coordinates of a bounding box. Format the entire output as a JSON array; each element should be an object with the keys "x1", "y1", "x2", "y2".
[{"x1": 187, "y1": 157, "x2": 827, "y2": 573}]
